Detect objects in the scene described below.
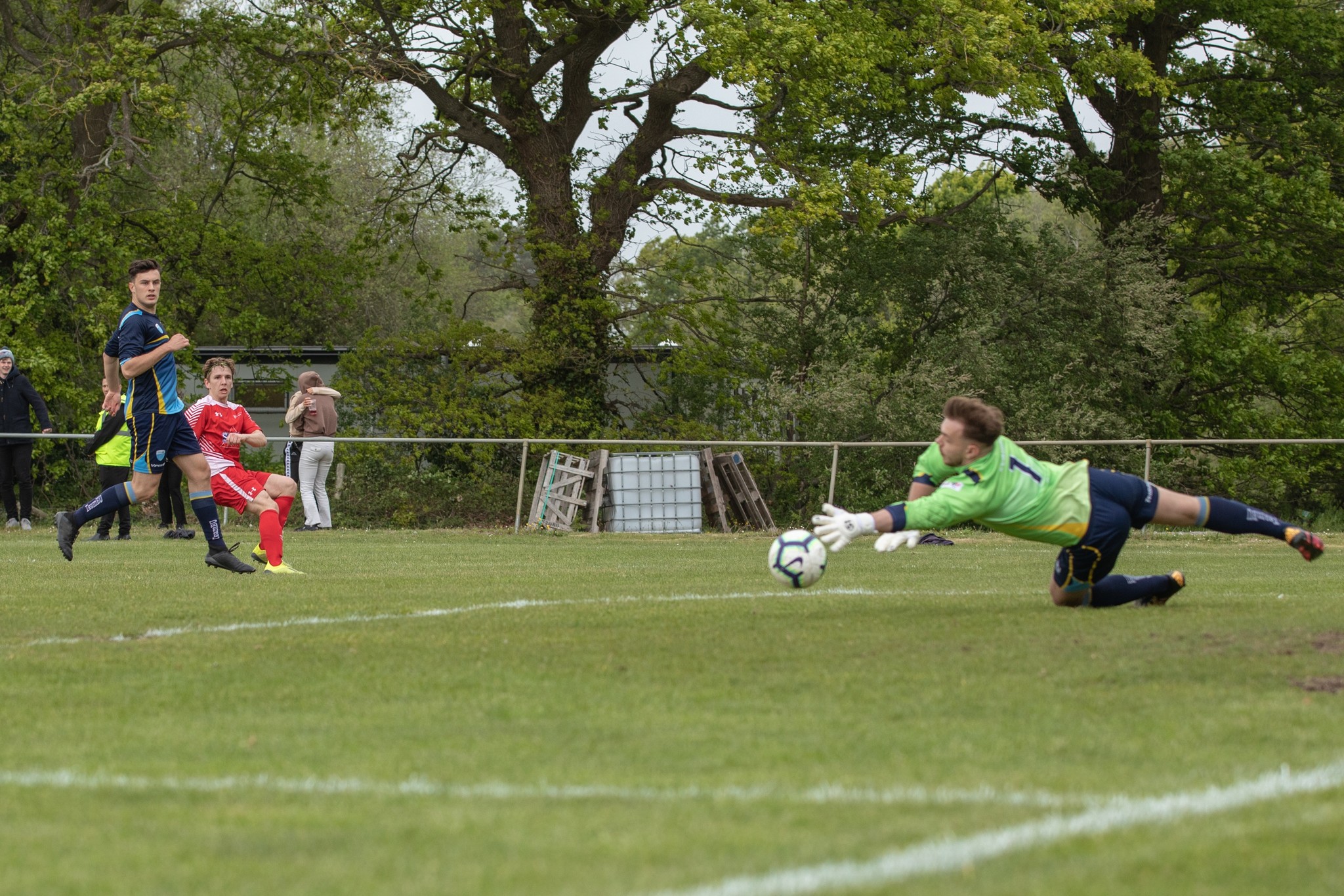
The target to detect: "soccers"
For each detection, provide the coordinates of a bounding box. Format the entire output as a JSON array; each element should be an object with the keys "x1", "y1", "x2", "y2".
[{"x1": 768, "y1": 529, "x2": 828, "y2": 588}]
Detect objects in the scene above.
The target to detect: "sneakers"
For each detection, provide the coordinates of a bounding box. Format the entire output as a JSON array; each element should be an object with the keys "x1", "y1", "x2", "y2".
[
  {"x1": 252, "y1": 544, "x2": 292, "y2": 569},
  {"x1": 205, "y1": 542, "x2": 256, "y2": 575},
  {"x1": 7, "y1": 518, "x2": 19, "y2": 528},
  {"x1": 295, "y1": 523, "x2": 319, "y2": 531},
  {"x1": 263, "y1": 559, "x2": 306, "y2": 575},
  {"x1": 1136, "y1": 571, "x2": 1186, "y2": 606},
  {"x1": 21, "y1": 518, "x2": 32, "y2": 530},
  {"x1": 53, "y1": 511, "x2": 80, "y2": 561},
  {"x1": 1284, "y1": 526, "x2": 1324, "y2": 563}
]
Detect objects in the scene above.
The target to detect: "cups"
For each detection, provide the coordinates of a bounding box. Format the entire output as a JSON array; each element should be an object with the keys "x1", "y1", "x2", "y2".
[{"x1": 307, "y1": 399, "x2": 317, "y2": 415}]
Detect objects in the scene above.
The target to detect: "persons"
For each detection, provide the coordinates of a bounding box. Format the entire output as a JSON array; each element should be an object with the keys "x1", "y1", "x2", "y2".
[
  {"x1": 173, "y1": 356, "x2": 306, "y2": 575},
  {"x1": 281, "y1": 384, "x2": 344, "y2": 514},
  {"x1": 810, "y1": 393, "x2": 1325, "y2": 608},
  {"x1": 156, "y1": 453, "x2": 187, "y2": 532},
  {"x1": 54, "y1": 254, "x2": 259, "y2": 574},
  {"x1": 292, "y1": 370, "x2": 337, "y2": 531},
  {"x1": 0, "y1": 346, "x2": 53, "y2": 530},
  {"x1": 84, "y1": 374, "x2": 133, "y2": 541}
]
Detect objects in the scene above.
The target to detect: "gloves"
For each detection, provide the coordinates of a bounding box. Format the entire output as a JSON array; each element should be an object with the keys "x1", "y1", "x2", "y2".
[
  {"x1": 874, "y1": 527, "x2": 921, "y2": 553},
  {"x1": 811, "y1": 502, "x2": 876, "y2": 552}
]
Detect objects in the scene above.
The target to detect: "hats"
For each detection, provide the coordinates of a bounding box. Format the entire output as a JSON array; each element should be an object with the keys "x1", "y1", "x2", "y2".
[{"x1": 0, "y1": 346, "x2": 15, "y2": 366}]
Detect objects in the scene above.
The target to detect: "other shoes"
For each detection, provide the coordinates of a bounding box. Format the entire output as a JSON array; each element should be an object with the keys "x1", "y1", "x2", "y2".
[
  {"x1": 115, "y1": 534, "x2": 131, "y2": 541},
  {"x1": 82, "y1": 534, "x2": 111, "y2": 541},
  {"x1": 176, "y1": 523, "x2": 184, "y2": 530},
  {"x1": 158, "y1": 522, "x2": 173, "y2": 529}
]
